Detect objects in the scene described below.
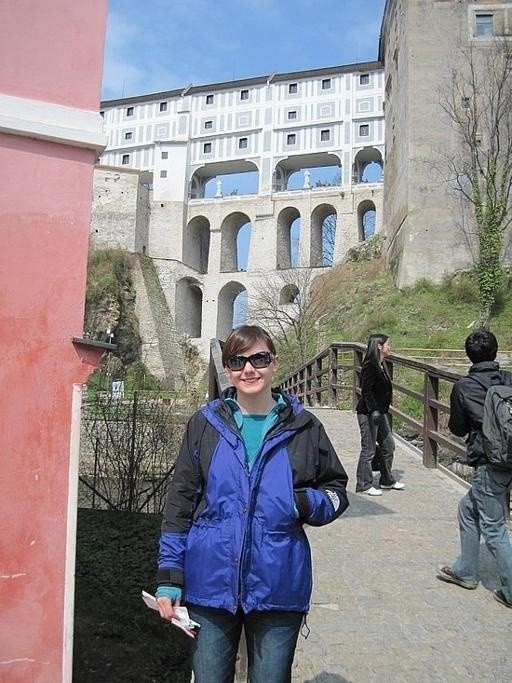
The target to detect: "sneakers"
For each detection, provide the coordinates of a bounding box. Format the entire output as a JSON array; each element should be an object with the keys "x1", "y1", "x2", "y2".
[
  {"x1": 362, "y1": 487, "x2": 383, "y2": 496},
  {"x1": 380, "y1": 481, "x2": 405, "y2": 490},
  {"x1": 437, "y1": 564, "x2": 477, "y2": 590},
  {"x1": 493, "y1": 590, "x2": 512, "y2": 609}
]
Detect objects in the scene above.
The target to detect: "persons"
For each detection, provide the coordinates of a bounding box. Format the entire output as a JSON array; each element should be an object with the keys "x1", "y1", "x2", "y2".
[
  {"x1": 155, "y1": 324, "x2": 349, "y2": 683},
  {"x1": 355, "y1": 333, "x2": 405, "y2": 496},
  {"x1": 435, "y1": 327, "x2": 512, "y2": 608}
]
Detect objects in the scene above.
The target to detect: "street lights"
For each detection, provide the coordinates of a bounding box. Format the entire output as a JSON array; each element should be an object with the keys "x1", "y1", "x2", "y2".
[{"x1": 106, "y1": 329, "x2": 114, "y2": 397}]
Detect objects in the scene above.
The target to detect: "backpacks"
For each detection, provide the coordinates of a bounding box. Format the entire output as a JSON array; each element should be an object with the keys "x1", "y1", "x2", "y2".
[{"x1": 465, "y1": 370, "x2": 512, "y2": 472}]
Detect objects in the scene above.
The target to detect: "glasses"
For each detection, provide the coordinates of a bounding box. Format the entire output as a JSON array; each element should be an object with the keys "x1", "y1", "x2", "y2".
[{"x1": 224, "y1": 351, "x2": 273, "y2": 372}]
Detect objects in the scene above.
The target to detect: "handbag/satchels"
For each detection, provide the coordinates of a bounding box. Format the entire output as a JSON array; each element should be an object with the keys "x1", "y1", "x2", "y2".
[{"x1": 372, "y1": 445, "x2": 383, "y2": 472}]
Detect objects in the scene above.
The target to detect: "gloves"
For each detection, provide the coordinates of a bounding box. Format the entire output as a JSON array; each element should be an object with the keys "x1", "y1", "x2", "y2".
[{"x1": 372, "y1": 410, "x2": 383, "y2": 426}]
